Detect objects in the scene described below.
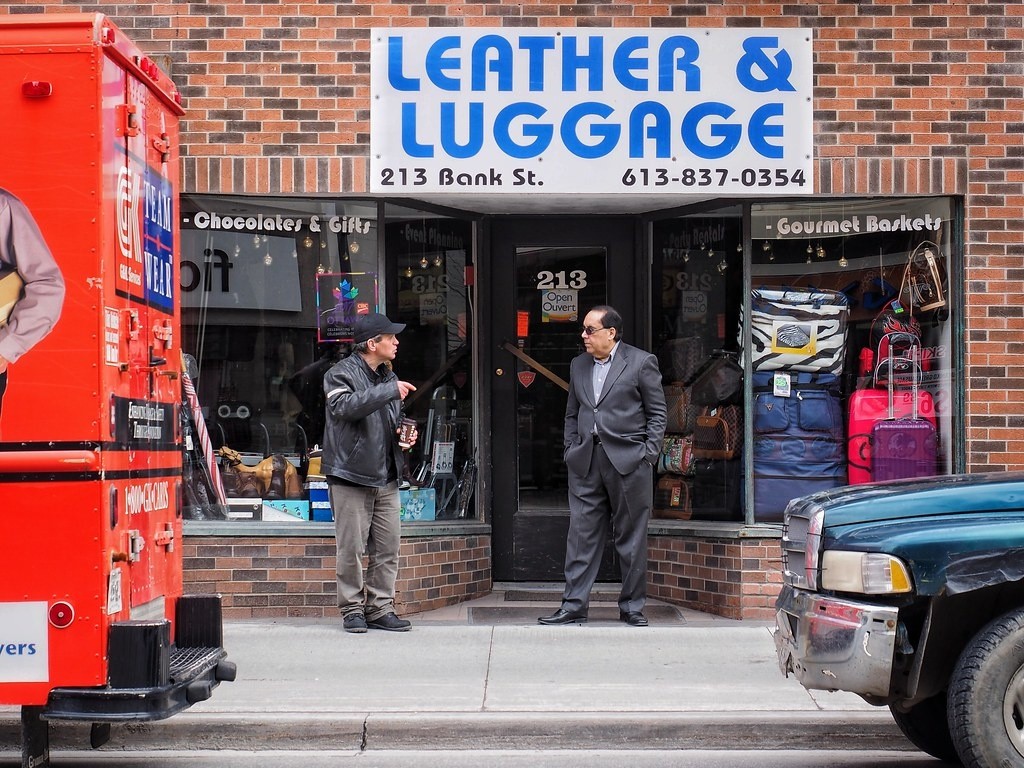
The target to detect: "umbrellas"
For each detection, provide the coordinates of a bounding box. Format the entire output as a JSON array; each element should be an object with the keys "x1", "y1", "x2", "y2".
[{"x1": 177, "y1": 348, "x2": 230, "y2": 522}]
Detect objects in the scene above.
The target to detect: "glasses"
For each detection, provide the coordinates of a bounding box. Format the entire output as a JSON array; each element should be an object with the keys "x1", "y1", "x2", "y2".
[{"x1": 579, "y1": 325, "x2": 606, "y2": 334}]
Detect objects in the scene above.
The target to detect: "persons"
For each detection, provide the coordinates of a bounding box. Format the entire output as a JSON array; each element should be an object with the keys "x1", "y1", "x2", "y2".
[
  {"x1": 536, "y1": 305, "x2": 669, "y2": 625},
  {"x1": 320, "y1": 311, "x2": 418, "y2": 633},
  {"x1": 287, "y1": 317, "x2": 354, "y2": 475},
  {"x1": 1, "y1": 187, "x2": 65, "y2": 412}
]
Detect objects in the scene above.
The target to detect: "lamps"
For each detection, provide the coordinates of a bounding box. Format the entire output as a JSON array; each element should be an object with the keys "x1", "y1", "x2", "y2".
[
  {"x1": 763, "y1": 203, "x2": 771, "y2": 252},
  {"x1": 682, "y1": 253, "x2": 689, "y2": 262},
  {"x1": 769, "y1": 202, "x2": 775, "y2": 262},
  {"x1": 349, "y1": 218, "x2": 360, "y2": 257},
  {"x1": 839, "y1": 196, "x2": 848, "y2": 267},
  {"x1": 320, "y1": 240, "x2": 327, "y2": 249},
  {"x1": 701, "y1": 242, "x2": 706, "y2": 251},
  {"x1": 816, "y1": 199, "x2": 826, "y2": 259},
  {"x1": 806, "y1": 254, "x2": 813, "y2": 264},
  {"x1": 708, "y1": 248, "x2": 714, "y2": 258},
  {"x1": 292, "y1": 232, "x2": 297, "y2": 257},
  {"x1": 343, "y1": 234, "x2": 349, "y2": 261},
  {"x1": 433, "y1": 220, "x2": 442, "y2": 266},
  {"x1": 736, "y1": 216, "x2": 743, "y2": 252},
  {"x1": 304, "y1": 225, "x2": 312, "y2": 248},
  {"x1": 262, "y1": 235, "x2": 267, "y2": 243},
  {"x1": 419, "y1": 210, "x2": 428, "y2": 269},
  {"x1": 807, "y1": 200, "x2": 814, "y2": 254},
  {"x1": 405, "y1": 223, "x2": 413, "y2": 278},
  {"x1": 317, "y1": 263, "x2": 325, "y2": 274},
  {"x1": 253, "y1": 229, "x2": 260, "y2": 249},
  {"x1": 264, "y1": 230, "x2": 272, "y2": 266},
  {"x1": 717, "y1": 259, "x2": 728, "y2": 276}
]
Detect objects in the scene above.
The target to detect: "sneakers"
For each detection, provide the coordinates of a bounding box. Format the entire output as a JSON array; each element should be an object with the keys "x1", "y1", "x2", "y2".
[
  {"x1": 342, "y1": 611, "x2": 369, "y2": 633},
  {"x1": 366, "y1": 610, "x2": 413, "y2": 632}
]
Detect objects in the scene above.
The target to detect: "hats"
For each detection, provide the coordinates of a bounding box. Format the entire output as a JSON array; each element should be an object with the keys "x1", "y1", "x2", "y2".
[{"x1": 353, "y1": 312, "x2": 407, "y2": 344}]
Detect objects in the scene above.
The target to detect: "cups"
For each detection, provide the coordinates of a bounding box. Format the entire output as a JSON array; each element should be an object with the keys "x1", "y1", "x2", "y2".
[{"x1": 398, "y1": 417, "x2": 417, "y2": 448}]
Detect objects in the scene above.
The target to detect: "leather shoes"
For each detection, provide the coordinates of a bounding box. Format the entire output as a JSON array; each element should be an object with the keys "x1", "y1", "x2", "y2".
[
  {"x1": 537, "y1": 609, "x2": 588, "y2": 624},
  {"x1": 619, "y1": 609, "x2": 649, "y2": 626}
]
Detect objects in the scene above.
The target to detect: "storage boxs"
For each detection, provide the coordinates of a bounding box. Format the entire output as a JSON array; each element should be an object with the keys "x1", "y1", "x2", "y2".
[
  {"x1": 739, "y1": 370, "x2": 844, "y2": 521},
  {"x1": 309, "y1": 481, "x2": 329, "y2": 501},
  {"x1": 399, "y1": 487, "x2": 435, "y2": 522},
  {"x1": 311, "y1": 501, "x2": 334, "y2": 522},
  {"x1": 263, "y1": 500, "x2": 310, "y2": 521},
  {"x1": 226, "y1": 498, "x2": 262, "y2": 520},
  {"x1": 737, "y1": 285, "x2": 850, "y2": 375}
]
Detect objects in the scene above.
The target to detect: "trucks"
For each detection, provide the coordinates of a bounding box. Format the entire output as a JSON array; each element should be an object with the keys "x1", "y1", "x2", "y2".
[{"x1": 0, "y1": 10, "x2": 239, "y2": 768}]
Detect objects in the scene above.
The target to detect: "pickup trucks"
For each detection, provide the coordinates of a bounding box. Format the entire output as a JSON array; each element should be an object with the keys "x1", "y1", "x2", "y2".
[{"x1": 772, "y1": 470, "x2": 1023, "y2": 766}]
